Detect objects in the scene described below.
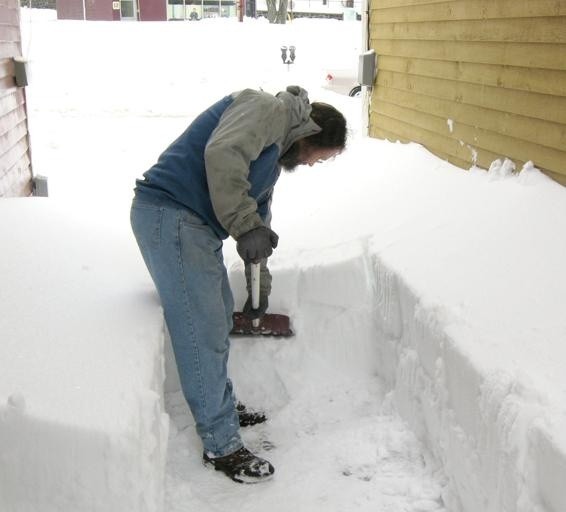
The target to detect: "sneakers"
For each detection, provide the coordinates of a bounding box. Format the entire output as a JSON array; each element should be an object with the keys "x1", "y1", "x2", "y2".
[
  {"x1": 236, "y1": 401, "x2": 267, "y2": 426},
  {"x1": 203, "y1": 446, "x2": 274, "y2": 483}
]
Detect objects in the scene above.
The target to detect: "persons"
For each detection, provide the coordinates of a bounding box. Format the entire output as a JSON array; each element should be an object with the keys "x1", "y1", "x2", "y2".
[
  {"x1": 131, "y1": 86, "x2": 346, "y2": 483},
  {"x1": 190, "y1": 8, "x2": 199, "y2": 21}
]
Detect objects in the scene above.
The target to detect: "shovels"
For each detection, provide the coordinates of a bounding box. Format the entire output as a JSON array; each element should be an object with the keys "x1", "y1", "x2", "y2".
[{"x1": 230, "y1": 260, "x2": 289, "y2": 338}]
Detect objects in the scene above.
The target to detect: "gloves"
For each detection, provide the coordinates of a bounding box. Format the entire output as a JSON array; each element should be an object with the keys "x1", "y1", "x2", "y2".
[
  {"x1": 236, "y1": 226, "x2": 278, "y2": 264},
  {"x1": 243, "y1": 294, "x2": 269, "y2": 320}
]
